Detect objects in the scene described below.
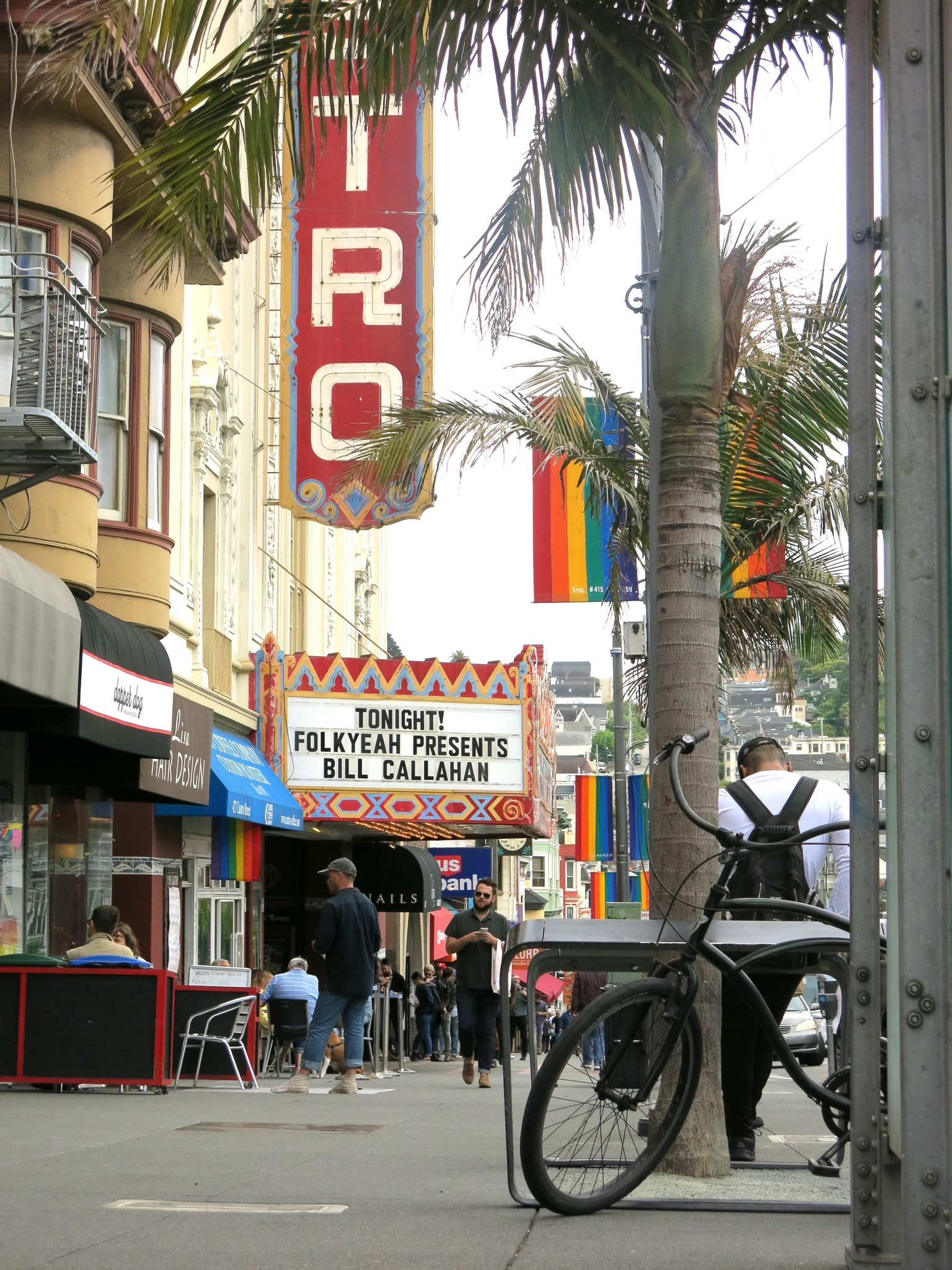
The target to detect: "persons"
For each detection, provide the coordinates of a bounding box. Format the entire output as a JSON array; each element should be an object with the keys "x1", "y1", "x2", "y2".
[
  {"x1": 715, "y1": 735, "x2": 851, "y2": 1162},
  {"x1": 62, "y1": 905, "x2": 606, "y2": 1095},
  {"x1": 271, "y1": 858, "x2": 381, "y2": 1094},
  {"x1": 444, "y1": 877, "x2": 510, "y2": 1088}
]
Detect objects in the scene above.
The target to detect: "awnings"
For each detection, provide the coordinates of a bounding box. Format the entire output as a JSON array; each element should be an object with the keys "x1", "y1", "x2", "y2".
[
  {"x1": 1, "y1": 548, "x2": 306, "y2": 834},
  {"x1": 513, "y1": 970, "x2": 563, "y2": 1002},
  {"x1": 352, "y1": 844, "x2": 444, "y2": 913}
]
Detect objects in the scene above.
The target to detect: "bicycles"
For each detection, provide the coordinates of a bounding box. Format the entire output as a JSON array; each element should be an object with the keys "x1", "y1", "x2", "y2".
[{"x1": 519, "y1": 726, "x2": 887, "y2": 1216}]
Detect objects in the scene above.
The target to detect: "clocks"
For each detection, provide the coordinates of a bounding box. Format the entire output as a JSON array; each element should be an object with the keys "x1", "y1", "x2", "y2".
[{"x1": 499, "y1": 838, "x2": 527, "y2": 851}]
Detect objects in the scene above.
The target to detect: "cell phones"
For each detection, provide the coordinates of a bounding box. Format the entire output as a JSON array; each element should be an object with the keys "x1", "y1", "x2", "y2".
[{"x1": 479, "y1": 927, "x2": 489, "y2": 938}]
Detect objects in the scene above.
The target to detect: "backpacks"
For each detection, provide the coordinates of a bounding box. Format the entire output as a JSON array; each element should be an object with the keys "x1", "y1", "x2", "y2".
[{"x1": 724, "y1": 776, "x2": 823, "y2": 969}]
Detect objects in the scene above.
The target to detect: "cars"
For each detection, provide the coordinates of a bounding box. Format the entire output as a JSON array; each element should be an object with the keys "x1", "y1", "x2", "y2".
[{"x1": 771, "y1": 992, "x2": 840, "y2": 1066}]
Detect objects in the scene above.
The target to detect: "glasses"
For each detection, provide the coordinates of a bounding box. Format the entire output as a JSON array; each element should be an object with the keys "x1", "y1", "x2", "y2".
[
  {"x1": 424, "y1": 973, "x2": 431, "y2": 976},
  {"x1": 86, "y1": 919, "x2": 93, "y2": 927},
  {"x1": 556, "y1": 1013, "x2": 560, "y2": 1015}
]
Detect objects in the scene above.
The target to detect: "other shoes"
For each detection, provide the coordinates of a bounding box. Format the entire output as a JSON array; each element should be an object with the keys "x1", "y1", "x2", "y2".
[
  {"x1": 520, "y1": 1056, "x2": 525, "y2": 1060},
  {"x1": 728, "y1": 1116, "x2": 764, "y2": 1160},
  {"x1": 594, "y1": 1066, "x2": 600, "y2": 1070},
  {"x1": 576, "y1": 1047, "x2": 579, "y2": 1057},
  {"x1": 479, "y1": 1073, "x2": 491, "y2": 1088},
  {"x1": 580, "y1": 1063, "x2": 591, "y2": 1069},
  {"x1": 410, "y1": 1053, "x2": 462, "y2": 1061},
  {"x1": 462, "y1": 1058, "x2": 473, "y2": 1085},
  {"x1": 511, "y1": 1055, "x2": 514, "y2": 1059},
  {"x1": 491, "y1": 1052, "x2": 502, "y2": 1068}
]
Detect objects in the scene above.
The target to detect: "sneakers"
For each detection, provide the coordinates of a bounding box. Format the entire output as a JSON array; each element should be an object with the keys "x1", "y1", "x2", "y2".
[
  {"x1": 271, "y1": 1073, "x2": 310, "y2": 1093},
  {"x1": 328, "y1": 1076, "x2": 358, "y2": 1093}
]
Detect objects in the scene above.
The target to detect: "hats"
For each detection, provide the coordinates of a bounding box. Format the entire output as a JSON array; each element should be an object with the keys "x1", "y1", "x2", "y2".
[
  {"x1": 317, "y1": 857, "x2": 357, "y2": 877},
  {"x1": 436, "y1": 963, "x2": 446, "y2": 969},
  {"x1": 737, "y1": 737, "x2": 785, "y2": 779}
]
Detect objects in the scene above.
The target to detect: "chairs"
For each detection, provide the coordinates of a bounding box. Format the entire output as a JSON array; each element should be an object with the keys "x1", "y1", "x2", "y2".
[{"x1": 174, "y1": 995, "x2": 374, "y2": 1093}]
